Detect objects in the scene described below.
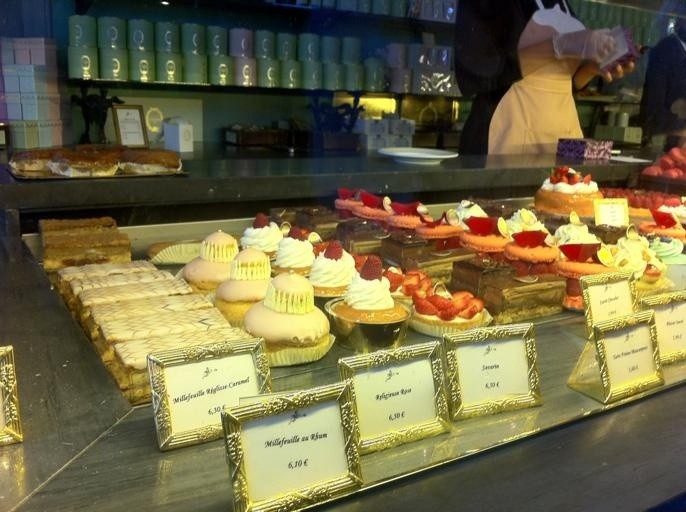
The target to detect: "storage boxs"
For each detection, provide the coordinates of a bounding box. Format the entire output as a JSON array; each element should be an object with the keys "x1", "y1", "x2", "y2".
[{"x1": 1, "y1": 36, "x2": 62, "y2": 151}]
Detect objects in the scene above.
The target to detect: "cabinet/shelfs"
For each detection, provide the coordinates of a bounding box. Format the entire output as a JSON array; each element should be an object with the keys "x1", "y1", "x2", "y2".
[{"x1": 66, "y1": 0, "x2": 463, "y2": 102}]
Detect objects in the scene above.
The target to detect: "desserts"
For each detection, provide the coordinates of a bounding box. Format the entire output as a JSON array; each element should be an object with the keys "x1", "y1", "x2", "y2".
[
  {"x1": 54, "y1": 259, "x2": 255, "y2": 404},
  {"x1": 301, "y1": 188, "x2": 685, "y2": 339},
  {"x1": 38, "y1": 216, "x2": 132, "y2": 283},
  {"x1": 240, "y1": 212, "x2": 412, "y2": 325}
]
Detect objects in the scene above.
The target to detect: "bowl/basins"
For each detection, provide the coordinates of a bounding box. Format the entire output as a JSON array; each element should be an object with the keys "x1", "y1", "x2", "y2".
[{"x1": 322, "y1": 295, "x2": 416, "y2": 352}]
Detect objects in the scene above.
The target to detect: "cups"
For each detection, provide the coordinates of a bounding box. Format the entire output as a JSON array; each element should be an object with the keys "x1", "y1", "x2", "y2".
[{"x1": 66, "y1": 10, "x2": 458, "y2": 96}]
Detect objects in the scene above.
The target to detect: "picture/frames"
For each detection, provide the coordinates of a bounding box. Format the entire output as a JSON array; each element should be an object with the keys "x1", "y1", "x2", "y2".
[{"x1": 110, "y1": 104, "x2": 149, "y2": 149}]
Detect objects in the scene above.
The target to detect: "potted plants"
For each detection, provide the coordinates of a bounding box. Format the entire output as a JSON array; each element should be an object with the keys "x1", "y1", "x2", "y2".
[{"x1": 69, "y1": 85, "x2": 126, "y2": 145}]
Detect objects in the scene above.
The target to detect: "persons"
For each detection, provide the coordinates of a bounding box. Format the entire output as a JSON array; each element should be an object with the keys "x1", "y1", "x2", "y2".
[
  {"x1": 640, "y1": 18, "x2": 686, "y2": 153},
  {"x1": 453, "y1": 0, "x2": 644, "y2": 153}
]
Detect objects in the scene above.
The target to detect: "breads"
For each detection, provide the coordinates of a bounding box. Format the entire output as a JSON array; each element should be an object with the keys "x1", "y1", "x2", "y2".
[
  {"x1": 11, "y1": 142, "x2": 182, "y2": 178},
  {"x1": 641, "y1": 142, "x2": 685, "y2": 181}
]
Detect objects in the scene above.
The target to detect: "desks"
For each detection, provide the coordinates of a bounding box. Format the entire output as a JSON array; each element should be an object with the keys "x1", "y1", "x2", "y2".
[{"x1": 1, "y1": 196, "x2": 686, "y2": 511}]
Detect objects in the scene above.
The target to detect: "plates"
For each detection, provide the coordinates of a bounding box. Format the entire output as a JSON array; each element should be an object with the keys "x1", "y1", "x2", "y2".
[{"x1": 376, "y1": 144, "x2": 459, "y2": 167}]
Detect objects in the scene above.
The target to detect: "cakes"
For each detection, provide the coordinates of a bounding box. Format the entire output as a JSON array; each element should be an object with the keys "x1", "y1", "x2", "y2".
[
  {"x1": 147, "y1": 227, "x2": 331, "y2": 354},
  {"x1": 534, "y1": 167, "x2": 605, "y2": 219}
]
